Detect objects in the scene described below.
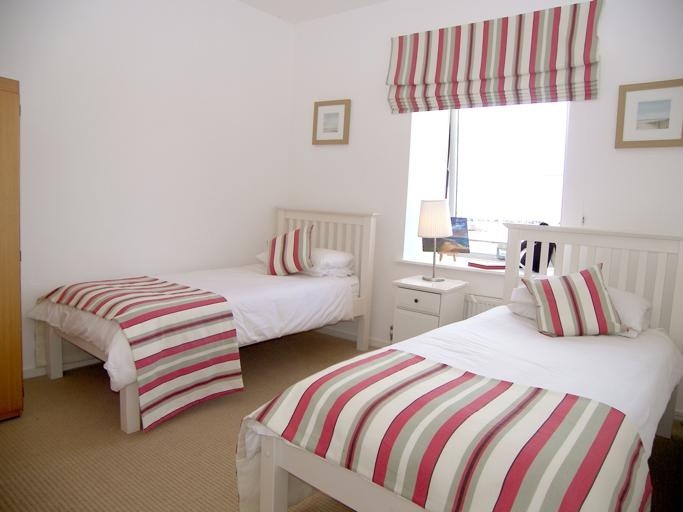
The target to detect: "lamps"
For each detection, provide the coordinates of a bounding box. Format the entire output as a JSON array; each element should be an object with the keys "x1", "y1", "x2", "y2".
[{"x1": 416, "y1": 197, "x2": 453, "y2": 284}]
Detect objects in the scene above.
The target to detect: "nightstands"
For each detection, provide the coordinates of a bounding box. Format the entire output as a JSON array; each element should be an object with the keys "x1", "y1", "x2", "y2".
[{"x1": 390, "y1": 273, "x2": 468, "y2": 343}]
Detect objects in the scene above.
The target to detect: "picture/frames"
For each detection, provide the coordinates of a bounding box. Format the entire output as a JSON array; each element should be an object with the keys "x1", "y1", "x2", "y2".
[
  {"x1": 311, "y1": 100, "x2": 350, "y2": 146},
  {"x1": 614, "y1": 77, "x2": 683, "y2": 147}
]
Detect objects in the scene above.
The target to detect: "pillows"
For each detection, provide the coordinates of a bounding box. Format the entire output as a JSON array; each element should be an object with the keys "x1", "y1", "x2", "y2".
[
  {"x1": 255, "y1": 224, "x2": 359, "y2": 279},
  {"x1": 505, "y1": 262, "x2": 653, "y2": 339}
]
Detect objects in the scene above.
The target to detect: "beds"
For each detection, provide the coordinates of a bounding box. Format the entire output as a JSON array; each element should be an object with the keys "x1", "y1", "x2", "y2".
[
  {"x1": 26, "y1": 206, "x2": 380, "y2": 435},
  {"x1": 232, "y1": 218, "x2": 683, "y2": 512}
]
